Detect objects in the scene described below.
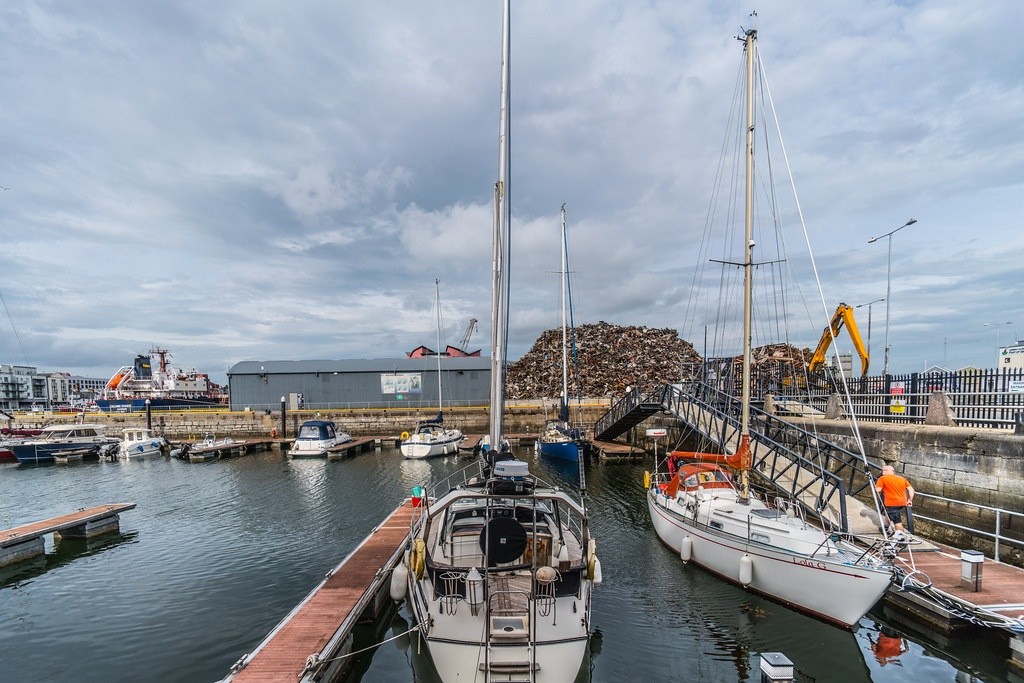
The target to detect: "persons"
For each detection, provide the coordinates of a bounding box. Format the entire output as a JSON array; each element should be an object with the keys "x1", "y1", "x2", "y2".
[{"x1": 874, "y1": 465, "x2": 915, "y2": 542}]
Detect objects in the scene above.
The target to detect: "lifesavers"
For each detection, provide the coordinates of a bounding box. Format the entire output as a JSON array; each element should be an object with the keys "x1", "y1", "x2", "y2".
[
  {"x1": 410, "y1": 539, "x2": 426, "y2": 580},
  {"x1": 643, "y1": 471, "x2": 650, "y2": 488},
  {"x1": 270, "y1": 429, "x2": 278, "y2": 437},
  {"x1": 700, "y1": 471, "x2": 715, "y2": 482},
  {"x1": 402, "y1": 431, "x2": 409, "y2": 439}
]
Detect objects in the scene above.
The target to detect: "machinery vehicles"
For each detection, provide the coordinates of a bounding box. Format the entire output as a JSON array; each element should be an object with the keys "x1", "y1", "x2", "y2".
[{"x1": 781, "y1": 300, "x2": 871, "y2": 398}]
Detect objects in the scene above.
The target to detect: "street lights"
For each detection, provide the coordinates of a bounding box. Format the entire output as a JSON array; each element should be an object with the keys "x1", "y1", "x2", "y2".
[
  {"x1": 866, "y1": 218, "x2": 918, "y2": 376},
  {"x1": 855, "y1": 298, "x2": 886, "y2": 354},
  {"x1": 983, "y1": 321, "x2": 1013, "y2": 370}
]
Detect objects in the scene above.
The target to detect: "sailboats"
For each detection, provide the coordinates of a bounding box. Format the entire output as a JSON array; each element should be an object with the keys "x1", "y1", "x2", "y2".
[
  {"x1": 641, "y1": 5, "x2": 911, "y2": 635},
  {"x1": 397, "y1": 279, "x2": 470, "y2": 460},
  {"x1": 298, "y1": 0, "x2": 608, "y2": 683},
  {"x1": 534, "y1": 200, "x2": 587, "y2": 464},
  {"x1": 472, "y1": 178, "x2": 516, "y2": 479}
]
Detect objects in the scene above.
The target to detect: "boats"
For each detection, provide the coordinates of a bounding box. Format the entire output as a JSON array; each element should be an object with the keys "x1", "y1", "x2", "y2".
[
  {"x1": 96, "y1": 427, "x2": 168, "y2": 463},
  {"x1": 288, "y1": 415, "x2": 354, "y2": 458},
  {"x1": 0, "y1": 409, "x2": 49, "y2": 464},
  {"x1": 2, "y1": 410, "x2": 124, "y2": 466},
  {"x1": 0, "y1": 343, "x2": 230, "y2": 414},
  {"x1": 169, "y1": 431, "x2": 238, "y2": 462}
]
